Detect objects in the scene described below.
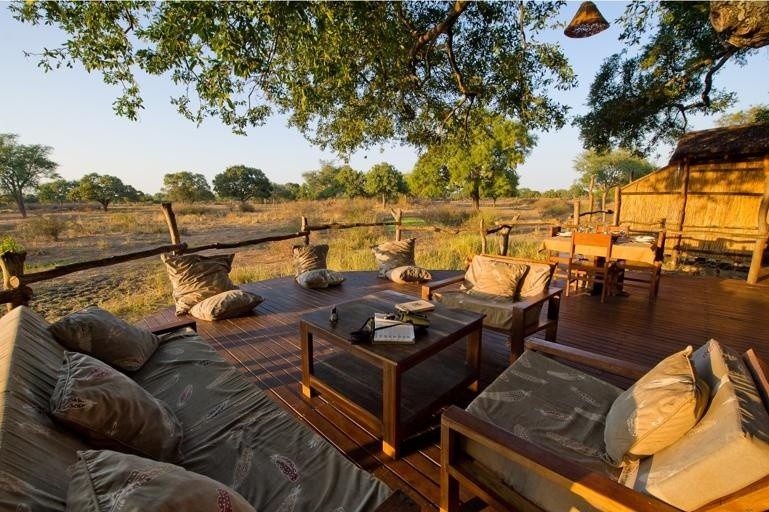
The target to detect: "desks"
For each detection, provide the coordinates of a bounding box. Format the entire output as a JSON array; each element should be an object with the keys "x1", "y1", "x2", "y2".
[{"x1": 293, "y1": 288, "x2": 489, "y2": 461}]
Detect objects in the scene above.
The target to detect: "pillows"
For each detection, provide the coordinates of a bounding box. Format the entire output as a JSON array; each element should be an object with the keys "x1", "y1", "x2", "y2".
[
  {"x1": 163, "y1": 236, "x2": 433, "y2": 324},
  {"x1": 464, "y1": 259, "x2": 529, "y2": 304},
  {"x1": 48, "y1": 347, "x2": 188, "y2": 458},
  {"x1": 602, "y1": 343, "x2": 712, "y2": 466},
  {"x1": 43, "y1": 302, "x2": 164, "y2": 373},
  {"x1": 62, "y1": 446, "x2": 259, "y2": 511}
]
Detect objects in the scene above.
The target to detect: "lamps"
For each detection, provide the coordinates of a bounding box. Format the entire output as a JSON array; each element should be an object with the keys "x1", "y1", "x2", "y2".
[{"x1": 562, "y1": 0, "x2": 611, "y2": 40}]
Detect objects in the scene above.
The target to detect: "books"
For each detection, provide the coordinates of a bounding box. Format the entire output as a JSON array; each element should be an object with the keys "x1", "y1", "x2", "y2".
[{"x1": 372, "y1": 298, "x2": 436, "y2": 345}]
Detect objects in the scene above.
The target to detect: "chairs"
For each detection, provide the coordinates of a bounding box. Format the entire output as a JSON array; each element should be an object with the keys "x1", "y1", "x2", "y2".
[
  {"x1": 538, "y1": 213, "x2": 669, "y2": 308},
  {"x1": 436, "y1": 336, "x2": 768, "y2": 512},
  {"x1": 421, "y1": 254, "x2": 564, "y2": 366}
]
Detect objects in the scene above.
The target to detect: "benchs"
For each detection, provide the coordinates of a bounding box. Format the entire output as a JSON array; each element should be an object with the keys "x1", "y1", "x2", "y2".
[{"x1": 2, "y1": 306, "x2": 424, "y2": 511}]
